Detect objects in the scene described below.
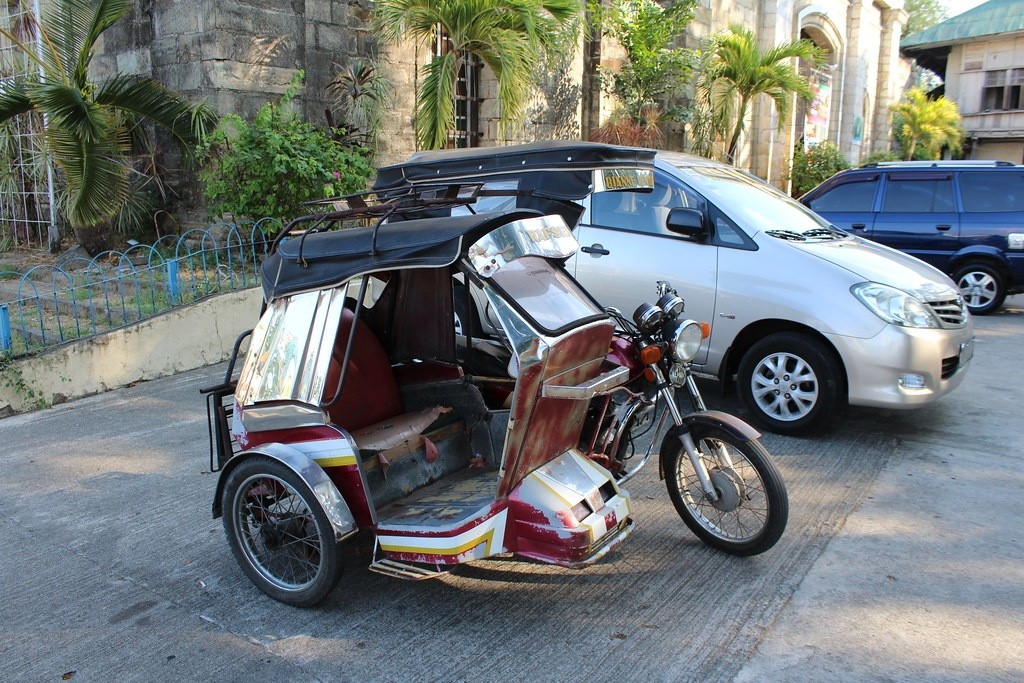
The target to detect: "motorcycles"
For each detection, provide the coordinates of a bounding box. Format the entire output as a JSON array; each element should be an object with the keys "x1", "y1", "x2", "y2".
[{"x1": 582, "y1": 279, "x2": 788, "y2": 559}]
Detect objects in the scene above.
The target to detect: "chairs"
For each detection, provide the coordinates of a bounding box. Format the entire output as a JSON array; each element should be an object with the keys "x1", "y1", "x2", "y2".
[
  {"x1": 591, "y1": 190, "x2": 638, "y2": 230},
  {"x1": 322, "y1": 305, "x2": 467, "y2": 473},
  {"x1": 644, "y1": 186, "x2": 681, "y2": 237}
]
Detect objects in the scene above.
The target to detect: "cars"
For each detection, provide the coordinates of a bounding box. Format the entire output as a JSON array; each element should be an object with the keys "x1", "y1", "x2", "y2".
[{"x1": 448, "y1": 144, "x2": 973, "y2": 439}]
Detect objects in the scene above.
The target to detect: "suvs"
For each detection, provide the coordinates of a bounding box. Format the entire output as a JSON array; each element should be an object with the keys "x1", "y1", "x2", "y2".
[{"x1": 794, "y1": 160, "x2": 1023, "y2": 317}]
